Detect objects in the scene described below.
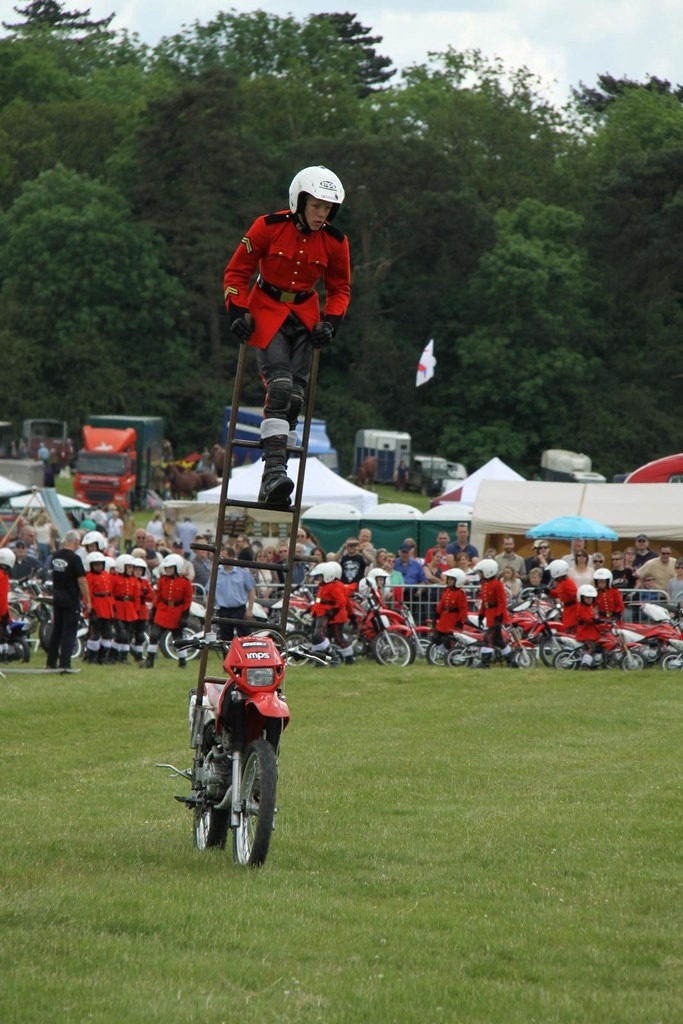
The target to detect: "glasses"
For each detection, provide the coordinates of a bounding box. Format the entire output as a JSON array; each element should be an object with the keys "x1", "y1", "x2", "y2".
[
  {"x1": 540, "y1": 539, "x2": 683, "y2": 570},
  {"x1": 348, "y1": 543, "x2": 357, "y2": 547},
  {"x1": 137, "y1": 537, "x2": 145, "y2": 539},
  {"x1": 16, "y1": 544, "x2": 25, "y2": 549},
  {"x1": 256, "y1": 529, "x2": 307, "y2": 561}
]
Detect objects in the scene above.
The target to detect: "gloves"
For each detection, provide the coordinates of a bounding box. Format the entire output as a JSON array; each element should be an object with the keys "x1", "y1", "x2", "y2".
[
  {"x1": 311, "y1": 320, "x2": 335, "y2": 349},
  {"x1": 430, "y1": 612, "x2": 621, "y2": 640},
  {"x1": 229, "y1": 307, "x2": 255, "y2": 344}
]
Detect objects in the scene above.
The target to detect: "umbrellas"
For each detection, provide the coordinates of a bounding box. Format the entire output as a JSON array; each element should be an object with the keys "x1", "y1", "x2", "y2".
[{"x1": 526, "y1": 513, "x2": 619, "y2": 557}]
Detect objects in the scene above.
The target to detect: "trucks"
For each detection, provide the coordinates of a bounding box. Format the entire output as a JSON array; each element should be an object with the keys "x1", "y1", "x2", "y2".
[{"x1": 223, "y1": 407, "x2": 340, "y2": 477}]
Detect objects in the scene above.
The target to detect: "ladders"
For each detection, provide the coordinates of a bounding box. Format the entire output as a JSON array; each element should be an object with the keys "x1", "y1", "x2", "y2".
[{"x1": 189, "y1": 314, "x2": 324, "y2": 748}]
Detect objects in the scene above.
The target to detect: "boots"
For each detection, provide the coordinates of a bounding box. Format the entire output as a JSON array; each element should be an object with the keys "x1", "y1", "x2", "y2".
[
  {"x1": 0, "y1": 637, "x2": 193, "y2": 670},
  {"x1": 471, "y1": 647, "x2": 520, "y2": 669},
  {"x1": 312, "y1": 637, "x2": 358, "y2": 666}
]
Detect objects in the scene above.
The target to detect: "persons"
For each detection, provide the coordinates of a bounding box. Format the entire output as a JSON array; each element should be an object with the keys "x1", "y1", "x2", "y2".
[
  {"x1": 8, "y1": 437, "x2": 74, "y2": 484},
  {"x1": 223, "y1": 166, "x2": 351, "y2": 502},
  {"x1": 395, "y1": 461, "x2": 410, "y2": 492},
  {"x1": 46, "y1": 530, "x2": 90, "y2": 675},
  {"x1": 0, "y1": 502, "x2": 683, "y2": 668},
  {"x1": 162, "y1": 438, "x2": 224, "y2": 489}
]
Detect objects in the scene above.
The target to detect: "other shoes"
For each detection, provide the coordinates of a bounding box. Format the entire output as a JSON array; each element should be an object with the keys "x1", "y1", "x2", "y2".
[{"x1": 257, "y1": 464, "x2": 295, "y2": 506}]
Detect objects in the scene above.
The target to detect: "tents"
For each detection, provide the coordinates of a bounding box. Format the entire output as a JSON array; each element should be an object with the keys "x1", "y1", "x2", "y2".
[
  {"x1": 420, "y1": 504, "x2": 473, "y2": 557},
  {"x1": 161, "y1": 501, "x2": 295, "y2": 545},
  {"x1": 430, "y1": 456, "x2": 528, "y2": 510},
  {"x1": 300, "y1": 503, "x2": 362, "y2": 554},
  {"x1": 197, "y1": 455, "x2": 377, "y2": 509},
  {"x1": 360, "y1": 504, "x2": 423, "y2": 558},
  {"x1": 470, "y1": 480, "x2": 683, "y2": 560}
]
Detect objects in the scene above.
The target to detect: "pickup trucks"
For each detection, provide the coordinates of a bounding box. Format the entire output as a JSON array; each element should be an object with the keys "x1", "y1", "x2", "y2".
[{"x1": 404, "y1": 455, "x2": 456, "y2": 495}]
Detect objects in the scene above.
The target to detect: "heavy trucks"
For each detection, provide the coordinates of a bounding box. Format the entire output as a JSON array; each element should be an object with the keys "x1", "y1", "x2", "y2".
[
  {"x1": 72, "y1": 414, "x2": 165, "y2": 512},
  {"x1": 0, "y1": 419, "x2": 73, "y2": 466}
]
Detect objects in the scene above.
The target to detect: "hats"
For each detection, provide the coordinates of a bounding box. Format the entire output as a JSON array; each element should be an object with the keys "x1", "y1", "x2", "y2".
[
  {"x1": 172, "y1": 539, "x2": 183, "y2": 548},
  {"x1": 399, "y1": 544, "x2": 412, "y2": 553},
  {"x1": 531, "y1": 540, "x2": 543, "y2": 550},
  {"x1": 636, "y1": 535, "x2": 649, "y2": 539}
]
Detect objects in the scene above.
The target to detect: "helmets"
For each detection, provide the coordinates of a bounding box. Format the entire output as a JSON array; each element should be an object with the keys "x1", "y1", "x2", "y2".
[
  {"x1": 359, "y1": 577, "x2": 377, "y2": 595},
  {"x1": 0, "y1": 548, "x2": 16, "y2": 569},
  {"x1": 443, "y1": 568, "x2": 467, "y2": 588},
  {"x1": 473, "y1": 559, "x2": 498, "y2": 579},
  {"x1": 367, "y1": 568, "x2": 390, "y2": 588},
  {"x1": 544, "y1": 559, "x2": 613, "y2": 603},
  {"x1": 310, "y1": 561, "x2": 343, "y2": 583},
  {"x1": 81, "y1": 531, "x2": 107, "y2": 550},
  {"x1": 289, "y1": 166, "x2": 345, "y2": 221},
  {"x1": 85, "y1": 551, "x2": 184, "y2": 576}
]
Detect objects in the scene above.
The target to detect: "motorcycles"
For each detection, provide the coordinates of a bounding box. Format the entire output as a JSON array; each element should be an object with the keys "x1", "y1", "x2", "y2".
[
  {"x1": 0, "y1": 571, "x2": 436, "y2": 668},
  {"x1": 427, "y1": 592, "x2": 683, "y2": 670},
  {"x1": 154, "y1": 632, "x2": 290, "y2": 870}
]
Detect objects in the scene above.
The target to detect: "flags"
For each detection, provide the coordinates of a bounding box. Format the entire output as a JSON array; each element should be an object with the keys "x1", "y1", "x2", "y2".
[{"x1": 415, "y1": 339, "x2": 437, "y2": 388}]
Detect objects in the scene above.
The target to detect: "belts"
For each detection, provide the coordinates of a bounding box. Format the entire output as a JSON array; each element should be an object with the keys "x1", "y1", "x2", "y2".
[{"x1": 256, "y1": 274, "x2": 315, "y2": 305}]
